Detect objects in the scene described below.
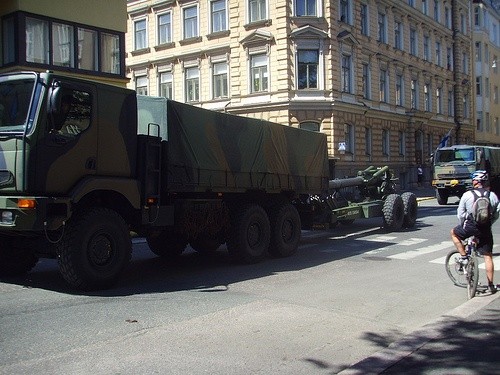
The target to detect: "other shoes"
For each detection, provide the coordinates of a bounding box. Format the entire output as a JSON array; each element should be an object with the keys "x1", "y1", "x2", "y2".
[
  {"x1": 488, "y1": 286, "x2": 497, "y2": 294},
  {"x1": 455, "y1": 256, "x2": 469, "y2": 265}
]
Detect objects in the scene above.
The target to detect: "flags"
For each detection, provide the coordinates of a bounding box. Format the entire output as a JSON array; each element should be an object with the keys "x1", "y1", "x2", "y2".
[{"x1": 437, "y1": 136, "x2": 449, "y2": 149}]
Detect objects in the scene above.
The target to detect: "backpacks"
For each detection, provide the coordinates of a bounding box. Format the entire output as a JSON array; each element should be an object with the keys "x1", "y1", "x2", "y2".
[{"x1": 469, "y1": 189, "x2": 492, "y2": 223}]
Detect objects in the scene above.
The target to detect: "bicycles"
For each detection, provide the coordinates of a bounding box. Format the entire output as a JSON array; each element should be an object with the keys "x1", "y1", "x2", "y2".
[{"x1": 445, "y1": 235, "x2": 480, "y2": 300}]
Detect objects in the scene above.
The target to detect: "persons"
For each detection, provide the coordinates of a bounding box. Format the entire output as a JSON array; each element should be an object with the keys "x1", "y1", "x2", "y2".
[
  {"x1": 451, "y1": 170, "x2": 500, "y2": 294},
  {"x1": 417, "y1": 165, "x2": 423, "y2": 185}
]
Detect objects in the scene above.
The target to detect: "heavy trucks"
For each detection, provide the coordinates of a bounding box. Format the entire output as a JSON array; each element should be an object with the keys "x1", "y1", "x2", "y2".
[{"x1": 0, "y1": 71, "x2": 332, "y2": 293}]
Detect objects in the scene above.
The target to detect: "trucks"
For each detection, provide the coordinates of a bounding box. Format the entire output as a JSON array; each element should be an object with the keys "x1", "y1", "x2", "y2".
[{"x1": 432, "y1": 145, "x2": 500, "y2": 205}]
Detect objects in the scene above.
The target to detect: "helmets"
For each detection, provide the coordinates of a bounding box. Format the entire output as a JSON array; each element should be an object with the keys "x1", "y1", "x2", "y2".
[{"x1": 470, "y1": 170, "x2": 488, "y2": 181}]
